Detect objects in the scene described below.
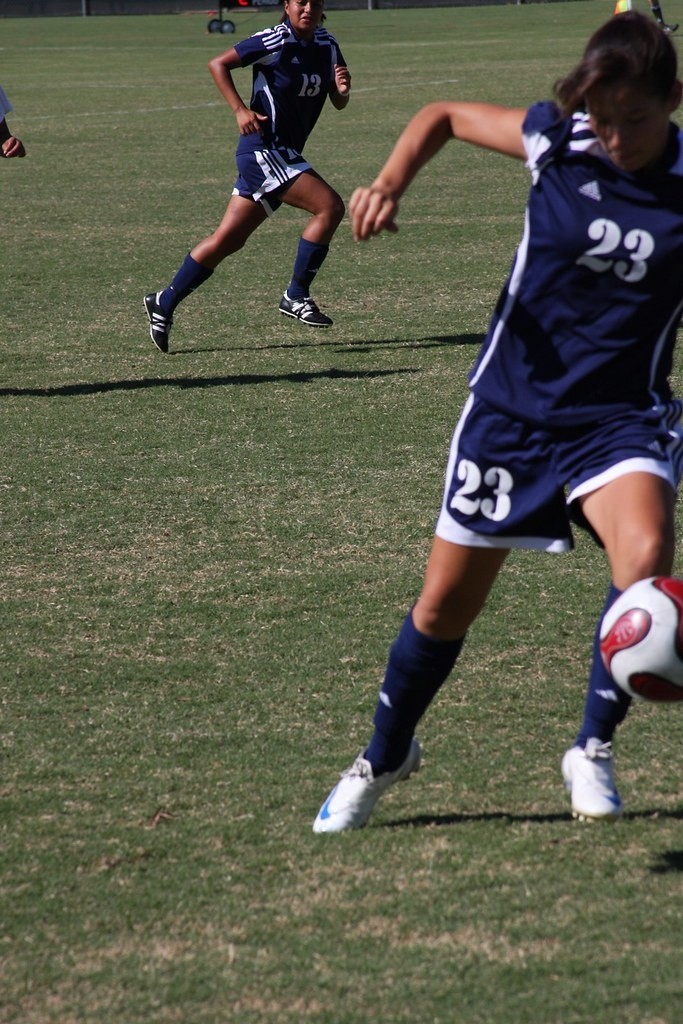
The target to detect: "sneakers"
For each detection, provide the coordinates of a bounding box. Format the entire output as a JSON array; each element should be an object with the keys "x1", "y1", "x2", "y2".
[
  {"x1": 278, "y1": 289, "x2": 333, "y2": 328},
  {"x1": 313, "y1": 739, "x2": 421, "y2": 833},
  {"x1": 561, "y1": 736, "x2": 622, "y2": 824},
  {"x1": 142, "y1": 291, "x2": 175, "y2": 353}
]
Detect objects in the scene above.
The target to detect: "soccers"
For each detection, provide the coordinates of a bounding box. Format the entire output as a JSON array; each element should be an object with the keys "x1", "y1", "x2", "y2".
[{"x1": 598, "y1": 574, "x2": 683, "y2": 703}]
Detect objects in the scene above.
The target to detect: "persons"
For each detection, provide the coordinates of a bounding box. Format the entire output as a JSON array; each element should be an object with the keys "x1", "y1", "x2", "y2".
[
  {"x1": 0, "y1": 85, "x2": 27, "y2": 159},
  {"x1": 311, "y1": 14, "x2": 683, "y2": 834},
  {"x1": 647, "y1": 0, "x2": 679, "y2": 35},
  {"x1": 143, "y1": 0, "x2": 352, "y2": 352}
]
